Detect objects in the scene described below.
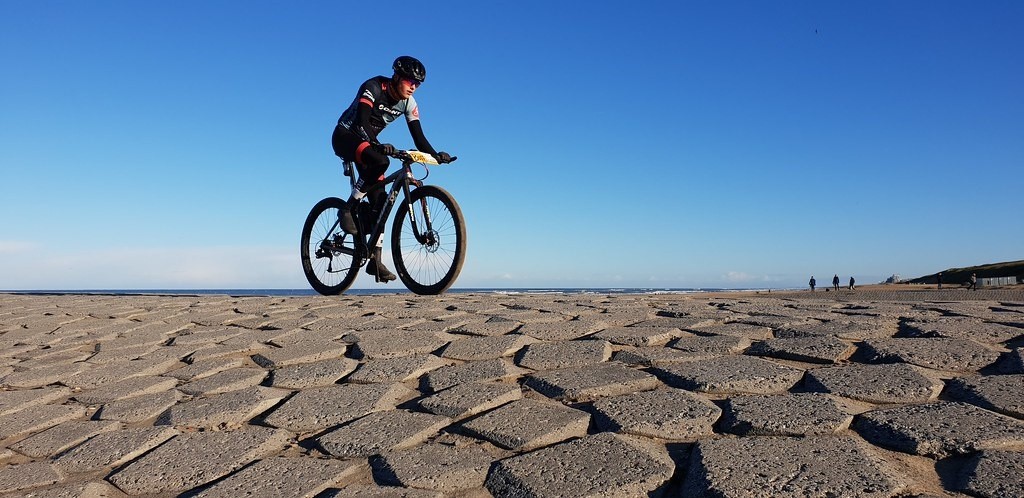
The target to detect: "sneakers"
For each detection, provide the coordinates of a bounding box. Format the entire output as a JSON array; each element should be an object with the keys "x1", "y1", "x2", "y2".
[
  {"x1": 365, "y1": 259, "x2": 396, "y2": 280},
  {"x1": 336, "y1": 207, "x2": 357, "y2": 235}
]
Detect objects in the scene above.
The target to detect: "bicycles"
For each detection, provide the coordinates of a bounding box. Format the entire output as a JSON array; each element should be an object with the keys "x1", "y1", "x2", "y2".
[{"x1": 301, "y1": 149, "x2": 466, "y2": 295}]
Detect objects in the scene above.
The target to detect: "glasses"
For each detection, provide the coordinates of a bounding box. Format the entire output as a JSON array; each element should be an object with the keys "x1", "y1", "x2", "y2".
[{"x1": 400, "y1": 76, "x2": 421, "y2": 88}]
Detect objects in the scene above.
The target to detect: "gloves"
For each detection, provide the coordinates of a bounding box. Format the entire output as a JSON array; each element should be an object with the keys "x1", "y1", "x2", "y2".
[
  {"x1": 379, "y1": 143, "x2": 393, "y2": 156},
  {"x1": 435, "y1": 151, "x2": 450, "y2": 163}
]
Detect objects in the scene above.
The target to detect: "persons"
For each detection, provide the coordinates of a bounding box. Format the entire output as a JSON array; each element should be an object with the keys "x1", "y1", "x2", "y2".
[
  {"x1": 848, "y1": 277, "x2": 856, "y2": 290},
  {"x1": 937, "y1": 273, "x2": 943, "y2": 291},
  {"x1": 809, "y1": 276, "x2": 816, "y2": 293},
  {"x1": 832, "y1": 274, "x2": 840, "y2": 291},
  {"x1": 966, "y1": 273, "x2": 977, "y2": 291},
  {"x1": 332, "y1": 55, "x2": 451, "y2": 283}
]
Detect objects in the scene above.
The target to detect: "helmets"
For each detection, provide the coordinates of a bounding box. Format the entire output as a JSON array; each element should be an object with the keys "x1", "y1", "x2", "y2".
[{"x1": 392, "y1": 56, "x2": 426, "y2": 82}]
